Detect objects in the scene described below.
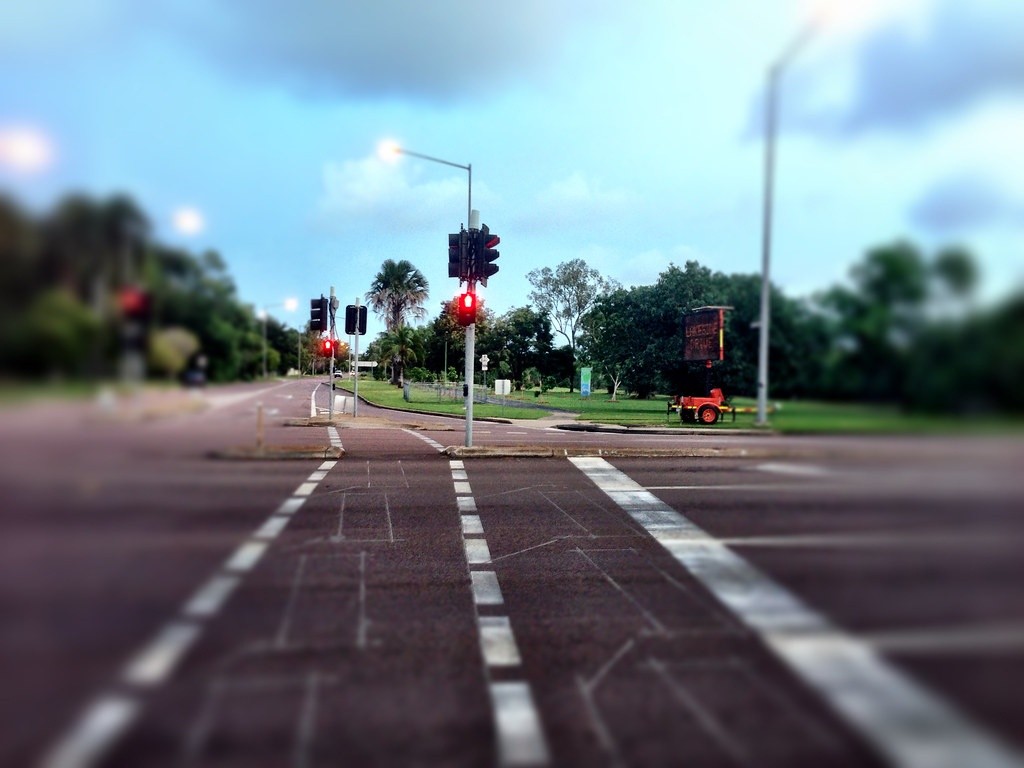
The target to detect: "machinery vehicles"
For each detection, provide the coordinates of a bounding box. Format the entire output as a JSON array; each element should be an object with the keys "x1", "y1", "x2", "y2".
[{"x1": 667, "y1": 387, "x2": 736, "y2": 425}]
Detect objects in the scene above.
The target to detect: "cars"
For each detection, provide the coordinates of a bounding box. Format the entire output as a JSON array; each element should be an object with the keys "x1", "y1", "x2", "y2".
[{"x1": 334, "y1": 370, "x2": 343, "y2": 378}]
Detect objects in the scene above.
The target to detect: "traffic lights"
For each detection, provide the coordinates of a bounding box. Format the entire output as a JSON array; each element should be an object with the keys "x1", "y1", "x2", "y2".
[
  {"x1": 458, "y1": 292, "x2": 476, "y2": 327},
  {"x1": 475, "y1": 223, "x2": 500, "y2": 287},
  {"x1": 323, "y1": 340, "x2": 332, "y2": 358},
  {"x1": 448, "y1": 223, "x2": 468, "y2": 287}
]
